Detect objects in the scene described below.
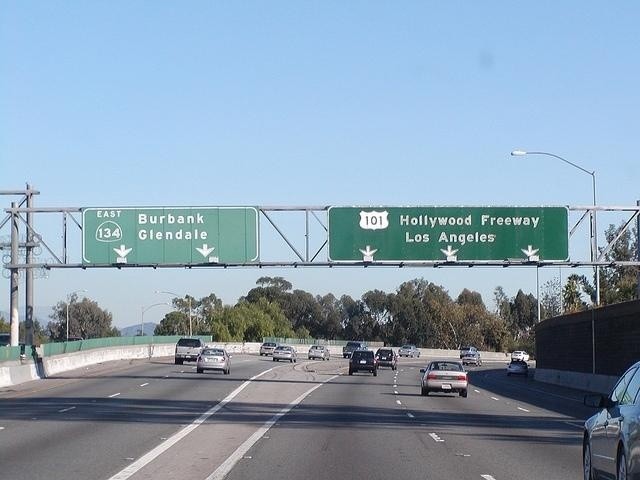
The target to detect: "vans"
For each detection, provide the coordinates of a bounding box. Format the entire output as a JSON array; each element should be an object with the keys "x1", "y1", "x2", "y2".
[{"x1": 68, "y1": 336, "x2": 83, "y2": 340}]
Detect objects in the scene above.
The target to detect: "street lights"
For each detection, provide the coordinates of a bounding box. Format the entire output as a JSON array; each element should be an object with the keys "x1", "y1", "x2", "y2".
[
  {"x1": 66, "y1": 288, "x2": 88, "y2": 341},
  {"x1": 509, "y1": 150, "x2": 600, "y2": 308},
  {"x1": 141, "y1": 300, "x2": 173, "y2": 337},
  {"x1": 156, "y1": 289, "x2": 193, "y2": 336}
]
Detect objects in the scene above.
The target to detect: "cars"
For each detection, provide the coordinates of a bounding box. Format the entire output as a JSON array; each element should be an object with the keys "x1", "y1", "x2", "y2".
[
  {"x1": 581, "y1": 360, "x2": 640, "y2": 480},
  {"x1": 506, "y1": 360, "x2": 531, "y2": 378},
  {"x1": 511, "y1": 350, "x2": 530, "y2": 362},
  {"x1": 307, "y1": 346, "x2": 330, "y2": 361},
  {"x1": 419, "y1": 360, "x2": 469, "y2": 399},
  {"x1": 398, "y1": 345, "x2": 421, "y2": 358},
  {"x1": 259, "y1": 342, "x2": 281, "y2": 356},
  {"x1": 195, "y1": 345, "x2": 232, "y2": 375},
  {"x1": 462, "y1": 352, "x2": 482, "y2": 366},
  {"x1": 272, "y1": 345, "x2": 297, "y2": 363},
  {"x1": 0, "y1": 332, "x2": 10, "y2": 348}
]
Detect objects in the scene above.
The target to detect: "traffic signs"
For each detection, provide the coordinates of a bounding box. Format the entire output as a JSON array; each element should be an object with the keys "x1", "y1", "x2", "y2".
[
  {"x1": 326, "y1": 205, "x2": 571, "y2": 267},
  {"x1": 80, "y1": 204, "x2": 261, "y2": 270}
]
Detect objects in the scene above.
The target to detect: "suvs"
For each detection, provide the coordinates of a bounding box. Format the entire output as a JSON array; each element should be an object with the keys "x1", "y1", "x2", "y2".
[
  {"x1": 343, "y1": 341, "x2": 367, "y2": 358},
  {"x1": 460, "y1": 347, "x2": 481, "y2": 359},
  {"x1": 173, "y1": 337, "x2": 208, "y2": 365},
  {"x1": 375, "y1": 348, "x2": 399, "y2": 371},
  {"x1": 348, "y1": 349, "x2": 378, "y2": 377}
]
